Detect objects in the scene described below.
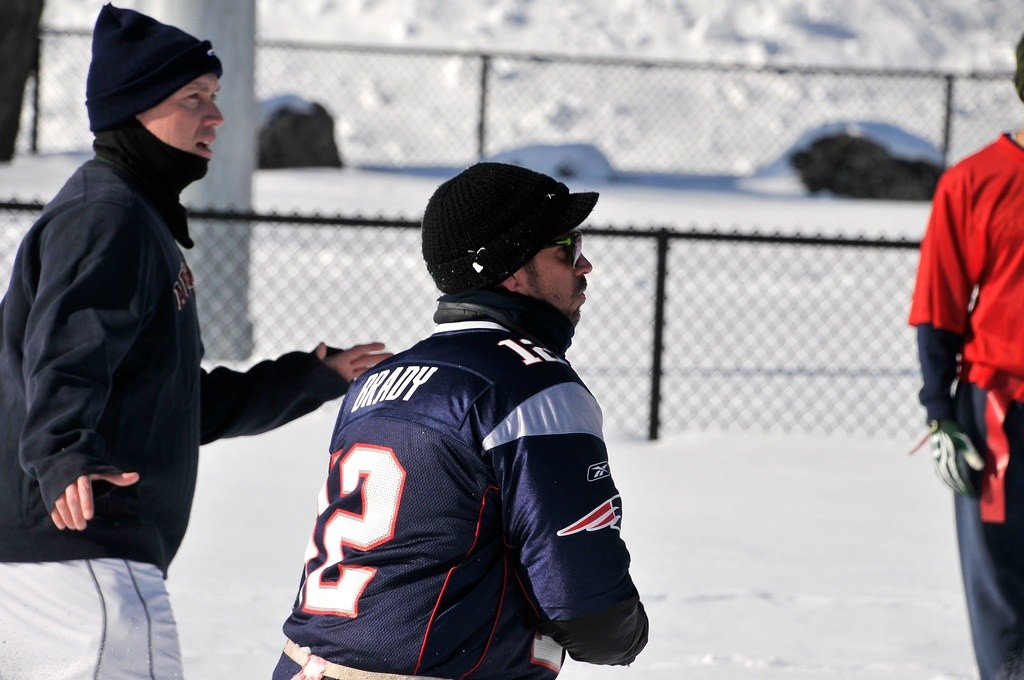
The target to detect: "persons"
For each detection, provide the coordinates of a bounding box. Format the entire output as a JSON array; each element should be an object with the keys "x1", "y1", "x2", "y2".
[
  {"x1": 908, "y1": 34, "x2": 1024, "y2": 680},
  {"x1": 0, "y1": 0, "x2": 45, "y2": 161},
  {"x1": 0, "y1": 4, "x2": 395, "y2": 680},
  {"x1": 273, "y1": 162, "x2": 649, "y2": 680}
]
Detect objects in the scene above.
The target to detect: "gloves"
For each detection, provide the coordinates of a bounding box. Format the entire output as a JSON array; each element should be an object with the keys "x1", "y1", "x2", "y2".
[{"x1": 925, "y1": 419, "x2": 982, "y2": 498}]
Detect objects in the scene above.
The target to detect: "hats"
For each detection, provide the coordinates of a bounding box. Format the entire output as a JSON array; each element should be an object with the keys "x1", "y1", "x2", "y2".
[
  {"x1": 422, "y1": 162, "x2": 600, "y2": 296},
  {"x1": 85, "y1": 6, "x2": 225, "y2": 133}
]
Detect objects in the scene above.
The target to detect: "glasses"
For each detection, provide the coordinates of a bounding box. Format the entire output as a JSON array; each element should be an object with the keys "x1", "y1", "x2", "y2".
[{"x1": 543, "y1": 229, "x2": 583, "y2": 269}]
